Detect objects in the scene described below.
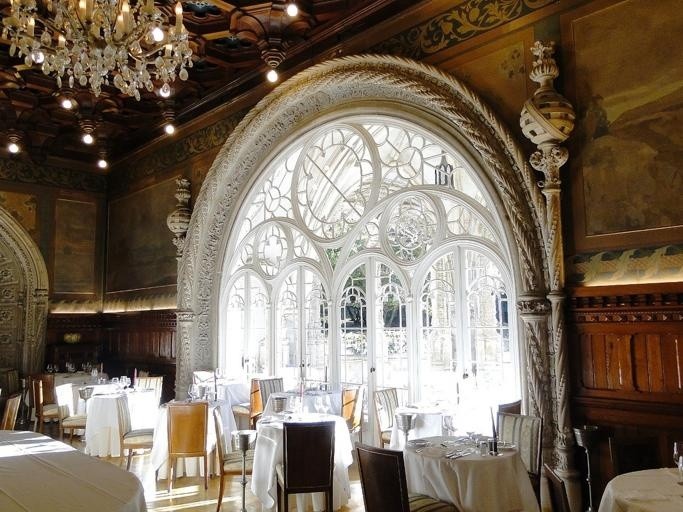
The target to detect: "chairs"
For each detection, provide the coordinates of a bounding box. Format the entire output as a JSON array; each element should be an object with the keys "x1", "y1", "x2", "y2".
[{"x1": 1, "y1": 367, "x2": 564, "y2": 512}]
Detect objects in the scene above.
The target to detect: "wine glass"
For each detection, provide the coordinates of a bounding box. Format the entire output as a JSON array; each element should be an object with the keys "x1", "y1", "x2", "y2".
[
  {"x1": 186, "y1": 383, "x2": 214, "y2": 403},
  {"x1": 672, "y1": 440, "x2": 683, "y2": 481},
  {"x1": 45, "y1": 361, "x2": 98, "y2": 378},
  {"x1": 95, "y1": 375, "x2": 131, "y2": 394}
]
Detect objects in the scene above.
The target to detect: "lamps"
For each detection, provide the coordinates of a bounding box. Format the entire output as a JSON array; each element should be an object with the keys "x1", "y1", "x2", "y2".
[
  {"x1": 2, "y1": 1, "x2": 191, "y2": 111},
  {"x1": 5, "y1": 126, "x2": 26, "y2": 155},
  {"x1": 157, "y1": 103, "x2": 180, "y2": 137},
  {"x1": 96, "y1": 140, "x2": 113, "y2": 169},
  {"x1": 272, "y1": 1, "x2": 297, "y2": 18},
  {"x1": 75, "y1": 112, "x2": 104, "y2": 148},
  {"x1": 257, "y1": 41, "x2": 286, "y2": 84}
]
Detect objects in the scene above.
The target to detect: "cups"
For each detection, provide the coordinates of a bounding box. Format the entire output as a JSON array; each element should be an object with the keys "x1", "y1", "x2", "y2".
[{"x1": 410, "y1": 431, "x2": 512, "y2": 458}]
